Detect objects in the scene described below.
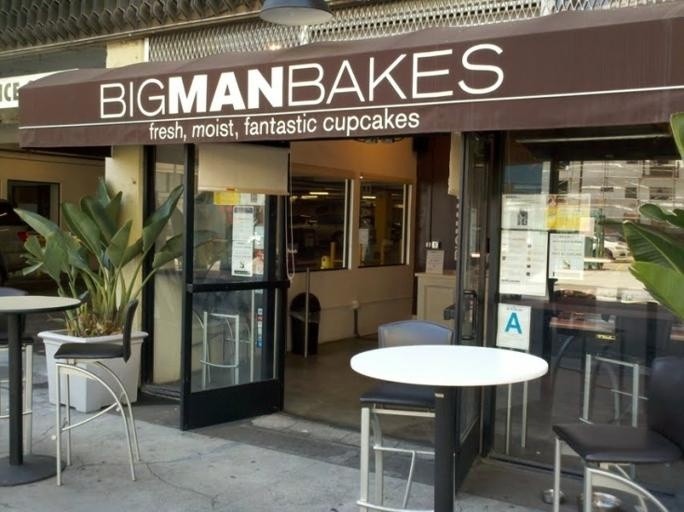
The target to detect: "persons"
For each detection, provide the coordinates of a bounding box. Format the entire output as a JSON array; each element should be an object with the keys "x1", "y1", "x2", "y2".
[{"x1": 330, "y1": 230, "x2": 342, "y2": 250}]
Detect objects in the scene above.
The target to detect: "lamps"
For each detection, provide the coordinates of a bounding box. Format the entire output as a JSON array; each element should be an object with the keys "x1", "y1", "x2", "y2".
[{"x1": 257, "y1": 0, "x2": 334, "y2": 26}]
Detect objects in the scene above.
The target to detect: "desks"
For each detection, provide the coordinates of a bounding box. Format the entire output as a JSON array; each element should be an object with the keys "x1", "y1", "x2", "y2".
[{"x1": 348, "y1": 345, "x2": 549, "y2": 512}]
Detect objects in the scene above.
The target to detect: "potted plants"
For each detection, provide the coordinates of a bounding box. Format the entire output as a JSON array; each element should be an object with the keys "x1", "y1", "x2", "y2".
[{"x1": 14, "y1": 177, "x2": 218, "y2": 414}]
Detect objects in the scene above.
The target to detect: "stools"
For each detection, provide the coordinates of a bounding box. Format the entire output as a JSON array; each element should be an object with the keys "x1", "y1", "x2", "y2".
[{"x1": 199, "y1": 307, "x2": 257, "y2": 392}]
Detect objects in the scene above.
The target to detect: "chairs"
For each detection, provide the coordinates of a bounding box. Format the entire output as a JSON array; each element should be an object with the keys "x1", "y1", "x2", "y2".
[
  {"x1": 357, "y1": 319, "x2": 454, "y2": 512},
  {"x1": 552, "y1": 356, "x2": 683, "y2": 511},
  {"x1": 0, "y1": 288, "x2": 144, "y2": 488}
]
